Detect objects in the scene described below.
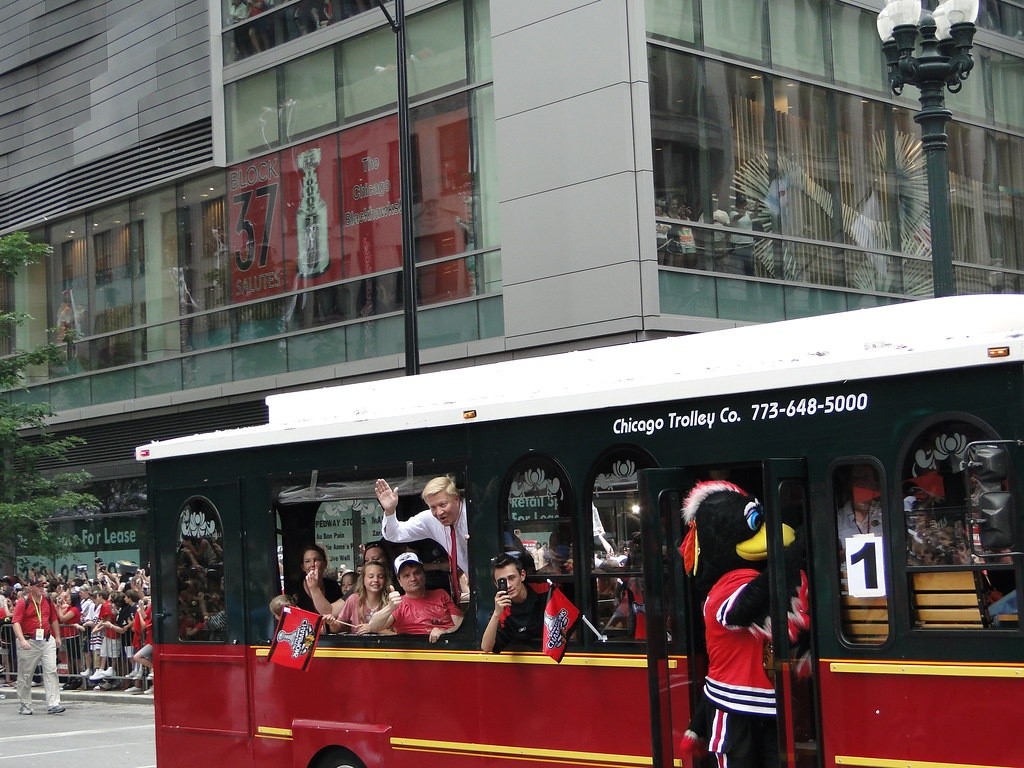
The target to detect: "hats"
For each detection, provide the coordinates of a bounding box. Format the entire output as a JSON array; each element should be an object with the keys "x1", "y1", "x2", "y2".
[
  {"x1": 31, "y1": 578, "x2": 47, "y2": 587},
  {"x1": 393, "y1": 552, "x2": 423, "y2": 577}
]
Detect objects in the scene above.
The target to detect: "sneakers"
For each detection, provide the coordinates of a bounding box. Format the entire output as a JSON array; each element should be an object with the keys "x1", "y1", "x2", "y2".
[
  {"x1": 124, "y1": 686, "x2": 143, "y2": 695},
  {"x1": 47, "y1": 705, "x2": 65, "y2": 713},
  {"x1": 80, "y1": 669, "x2": 92, "y2": 676},
  {"x1": 19, "y1": 707, "x2": 33, "y2": 714},
  {"x1": 89, "y1": 669, "x2": 106, "y2": 680},
  {"x1": 100, "y1": 667, "x2": 116, "y2": 676}
]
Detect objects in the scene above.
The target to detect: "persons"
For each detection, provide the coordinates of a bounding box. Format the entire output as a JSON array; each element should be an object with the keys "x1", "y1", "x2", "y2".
[
  {"x1": 270, "y1": 476, "x2": 672, "y2": 645},
  {"x1": 176, "y1": 519, "x2": 228, "y2": 643},
  {"x1": 656, "y1": 192, "x2": 765, "y2": 299},
  {"x1": 0, "y1": 556, "x2": 154, "y2": 697},
  {"x1": 12, "y1": 574, "x2": 66, "y2": 714},
  {"x1": 837, "y1": 462, "x2": 884, "y2": 596},
  {"x1": 903, "y1": 492, "x2": 1014, "y2": 566}
]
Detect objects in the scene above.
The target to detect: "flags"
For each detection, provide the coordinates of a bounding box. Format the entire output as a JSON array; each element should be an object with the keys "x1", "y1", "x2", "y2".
[
  {"x1": 542, "y1": 582, "x2": 583, "y2": 663},
  {"x1": 615, "y1": 580, "x2": 648, "y2": 640},
  {"x1": 266, "y1": 605, "x2": 325, "y2": 672}
]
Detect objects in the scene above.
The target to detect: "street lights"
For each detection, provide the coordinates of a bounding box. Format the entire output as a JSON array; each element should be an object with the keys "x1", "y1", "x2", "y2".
[{"x1": 875, "y1": 0, "x2": 979, "y2": 298}]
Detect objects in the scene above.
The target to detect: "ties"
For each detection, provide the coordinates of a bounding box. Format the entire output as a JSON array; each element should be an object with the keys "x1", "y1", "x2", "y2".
[{"x1": 450, "y1": 524, "x2": 461, "y2": 605}]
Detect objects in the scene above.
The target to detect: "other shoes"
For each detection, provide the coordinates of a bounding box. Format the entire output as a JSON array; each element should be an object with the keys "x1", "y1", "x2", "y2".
[
  {"x1": 125, "y1": 671, "x2": 137, "y2": 678},
  {"x1": 144, "y1": 685, "x2": 154, "y2": 694},
  {"x1": 31, "y1": 681, "x2": 41, "y2": 686},
  {"x1": 148, "y1": 668, "x2": 154, "y2": 677},
  {"x1": 132, "y1": 670, "x2": 144, "y2": 679}
]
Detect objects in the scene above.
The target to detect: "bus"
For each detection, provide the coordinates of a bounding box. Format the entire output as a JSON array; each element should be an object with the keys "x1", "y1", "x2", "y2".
[{"x1": 134, "y1": 295, "x2": 1024, "y2": 768}]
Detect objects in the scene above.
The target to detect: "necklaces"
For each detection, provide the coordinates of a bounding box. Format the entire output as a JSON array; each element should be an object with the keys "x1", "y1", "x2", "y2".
[{"x1": 852, "y1": 506, "x2": 870, "y2": 534}]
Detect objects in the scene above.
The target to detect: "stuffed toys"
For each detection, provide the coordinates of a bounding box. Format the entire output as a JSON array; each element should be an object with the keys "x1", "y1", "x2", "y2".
[{"x1": 680, "y1": 479, "x2": 811, "y2": 768}]
